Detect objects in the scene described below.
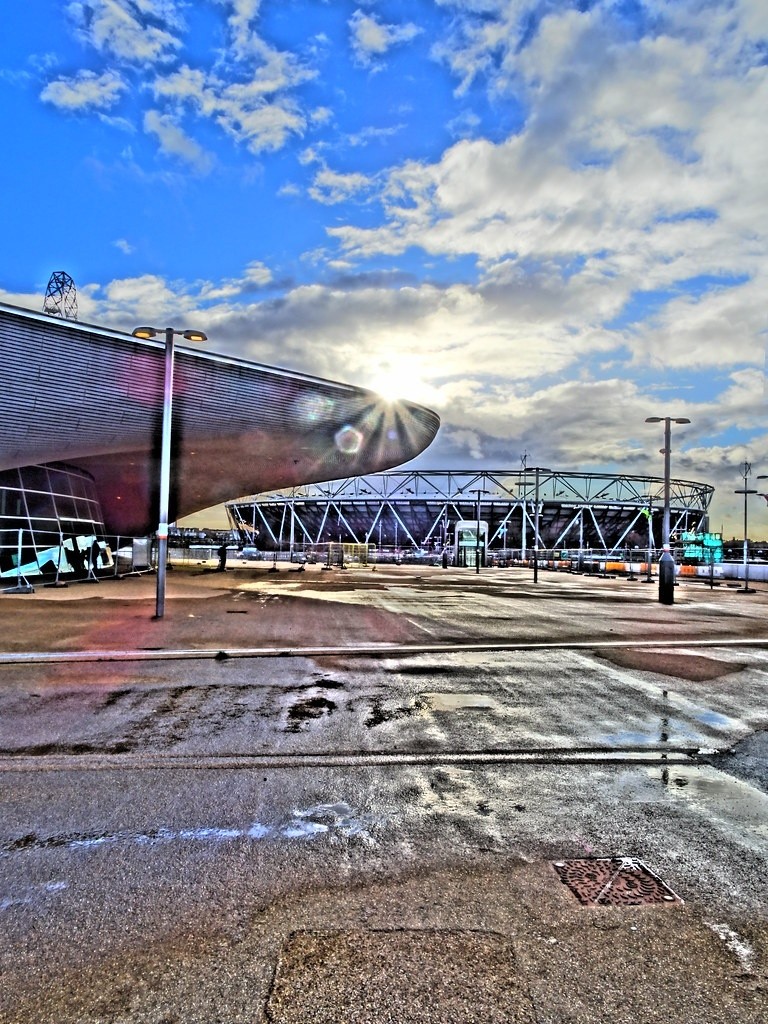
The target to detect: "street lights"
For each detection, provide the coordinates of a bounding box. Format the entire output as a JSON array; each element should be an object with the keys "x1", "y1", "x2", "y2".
[
  {"x1": 646, "y1": 416, "x2": 691, "y2": 603},
  {"x1": 499, "y1": 520, "x2": 511, "y2": 566},
  {"x1": 574, "y1": 507, "x2": 592, "y2": 572},
  {"x1": 469, "y1": 489, "x2": 490, "y2": 573},
  {"x1": 641, "y1": 495, "x2": 661, "y2": 583},
  {"x1": 132, "y1": 326, "x2": 209, "y2": 617},
  {"x1": 515, "y1": 482, "x2": 535, "y2": 559},
  {"x1": 524, "y1": 467, "x2": 550, "y2": 582},
  {"x1": 734, "y1": 490, "x2": 758, "y2": 594}
]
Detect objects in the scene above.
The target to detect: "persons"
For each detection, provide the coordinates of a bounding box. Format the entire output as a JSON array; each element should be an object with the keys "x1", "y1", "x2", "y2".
[
  {"x1": 690, "y1": 522, "x2": 697, "y2": 535},
  {"x1": 450, "y1": 551, "x2": 454, "y2": 565},
  {"x1": 218, "y1": 543, "x2": 228, "y2": 572},
  {"x1": 290, "y1": 545, "x2": 295, "y2": 563}
]
